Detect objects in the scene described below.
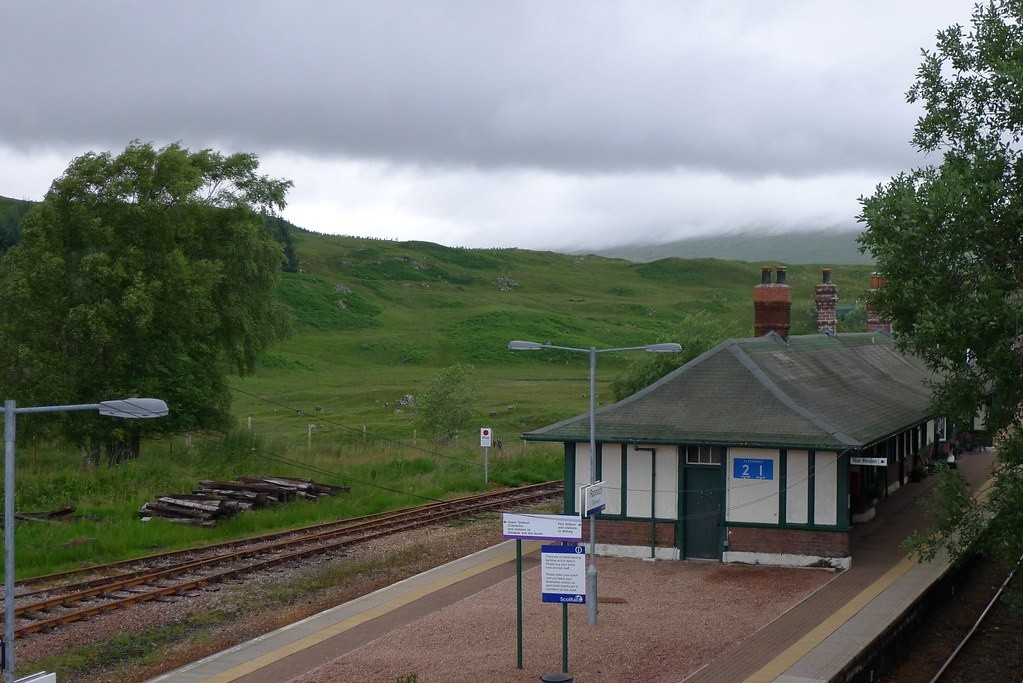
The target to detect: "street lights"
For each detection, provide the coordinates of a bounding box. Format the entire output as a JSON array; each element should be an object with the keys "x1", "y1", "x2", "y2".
[
  {"x1": 506, "y1": 340, "x2": 684, "y2": 625},
  {"x1": 0, "y1": 397, "x2": 169, "y2": 683}
]
